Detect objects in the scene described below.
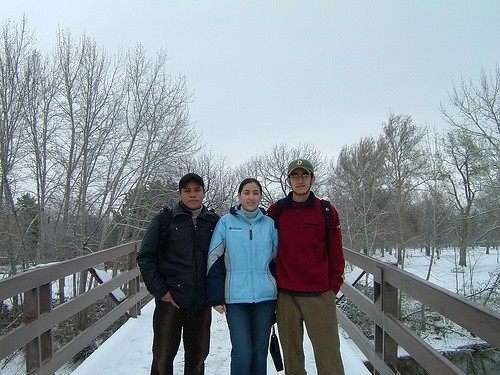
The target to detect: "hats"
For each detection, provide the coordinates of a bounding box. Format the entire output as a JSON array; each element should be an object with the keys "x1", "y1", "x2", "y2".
[
  {"x1": 287, "y1": 160, "x2": 314, "y2": 176},
  {"x1": 180, "y1": 173, "x2": 204, "y2": 190}
]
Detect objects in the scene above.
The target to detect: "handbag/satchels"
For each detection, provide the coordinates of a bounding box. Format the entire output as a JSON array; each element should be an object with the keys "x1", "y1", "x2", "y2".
[{"x1": 270, "y1": 325, "x2": 284, "y2": 372}]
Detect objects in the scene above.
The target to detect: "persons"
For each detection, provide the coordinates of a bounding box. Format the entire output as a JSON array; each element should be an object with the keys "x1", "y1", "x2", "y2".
[
  {"x1": 205, "y1": 178, "x2": 278, "y2": 375},
  {"x1": 266, "y1": 159, "x2": 346, "y2": 375},
  {"x1": 137, "y1": 173, "x2": 220, "y2": 375}
]
